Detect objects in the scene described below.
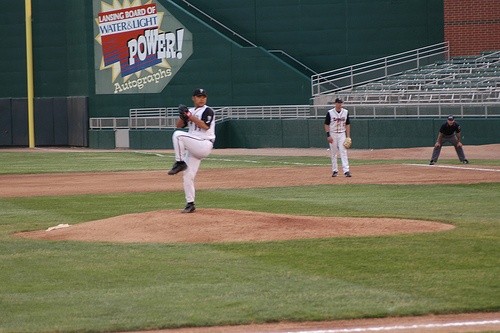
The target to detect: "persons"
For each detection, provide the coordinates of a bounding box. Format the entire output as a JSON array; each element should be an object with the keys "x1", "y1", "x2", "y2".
[
  {"x1": 429, "y1": 116, "x2": 468, "y2": 165},
  {"x1": 168, "y1": 88, "x2": 215, "y2": 213},
  {"x1": 324, "y1": 98, "x2": 352, "y2": 177}
]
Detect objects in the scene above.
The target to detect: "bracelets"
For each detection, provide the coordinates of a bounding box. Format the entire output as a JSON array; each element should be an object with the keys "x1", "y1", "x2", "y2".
[{"x1": 327, "y1": 132, "x2": 330, "y2": 137}]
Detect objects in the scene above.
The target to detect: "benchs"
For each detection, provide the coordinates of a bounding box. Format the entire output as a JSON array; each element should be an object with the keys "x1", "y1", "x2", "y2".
[{"x1": 335, "y1": 51, "x2": 500, "y2": 103}]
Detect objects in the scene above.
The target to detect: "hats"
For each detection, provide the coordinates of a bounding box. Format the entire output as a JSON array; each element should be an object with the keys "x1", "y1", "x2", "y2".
[
  {"x1": 193, "y1": 88, "x2": 208, "y2": 97},
  {"x1": 448, "y1": 116, "x2": 454, "y2": 120},
  {"x1": 335, "y1": 97, "x2": 342, "y2": 103}
]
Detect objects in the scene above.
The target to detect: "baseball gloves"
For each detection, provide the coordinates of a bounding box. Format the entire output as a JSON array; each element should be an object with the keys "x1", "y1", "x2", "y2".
[
  {"x1": 343, "y1": 138, "x2": 352, "y2": 149},
  {"x1": 178, "y1": 104, "x2": 189, "y2": 121}
]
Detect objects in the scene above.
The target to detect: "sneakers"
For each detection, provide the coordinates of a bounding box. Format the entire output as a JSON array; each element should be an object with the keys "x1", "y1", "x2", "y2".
[
  {"x1": 182, "y1": 203, "x2": 195, "y2": 213},
  {"x1": 345, "y1": 172, "x2": 351, "y2": 177},
  {"x1": 168, "y1": 161, "x2": 188, "y2": 175},
  {"x1": 332, "y1": 171, "x2": 338, "y2": 177}
]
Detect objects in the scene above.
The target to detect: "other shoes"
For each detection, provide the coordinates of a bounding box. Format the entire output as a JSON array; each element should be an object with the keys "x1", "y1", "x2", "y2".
[
  {"x1": 462, "y1": 160, "x2": 468, "y2": 164},
  {"x1": 430, "y1": 161, "x2": 435, "y2": 165}
]
